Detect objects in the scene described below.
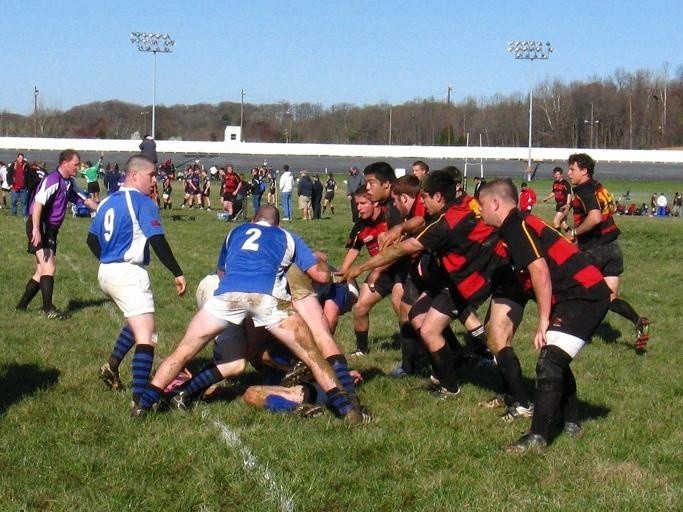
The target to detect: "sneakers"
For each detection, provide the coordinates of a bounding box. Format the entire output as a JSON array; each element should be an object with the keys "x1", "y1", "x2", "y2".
[
  {"x1": 291, "y1": 404, "x2": 322, "y2": 417},
  {"x1": 97, "y1": 361, "x2": 125, "y2": 393},
  {"x1": 390, "y1": 366, "x2": 460, "y2": 400},
  {"x1": 343, "y1": 413, "x2": 371, "y2": 427},
  {"x1": 129, "y1": 389, "x2": 191, "y2": 418},
  {"x1": 479, "y1": 393, "x2": 583, "y2": 454},
  {"x1": 282, "y1": 362, "x2": 314, "y2": 386},
  {"x1": 635, "y1": 317, "x2": 650, "y2": 351},
  {"x1": 44, "y1": 308, "x2": 71, "y2": 320}
]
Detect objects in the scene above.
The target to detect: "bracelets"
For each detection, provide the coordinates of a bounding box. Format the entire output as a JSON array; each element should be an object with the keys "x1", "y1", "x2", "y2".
[{"x1": 573, "y1": 230, "x2": 578, "y2": 238}]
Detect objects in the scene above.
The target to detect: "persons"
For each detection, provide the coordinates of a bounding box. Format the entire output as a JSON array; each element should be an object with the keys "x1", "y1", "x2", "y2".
[
  {"x1": 0, "y1": 134, "x2": 651, "y2": 457},
  {"x1": 651, "y1": 192, "x2": 683, "y2": 217}
]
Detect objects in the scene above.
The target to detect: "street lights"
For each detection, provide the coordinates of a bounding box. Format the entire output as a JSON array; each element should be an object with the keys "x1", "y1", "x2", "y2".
[
  {"x1": 445, "y1": 84, "x2": 452, "y2": 145},
  {"x1": 583, "y1": 119, "x2": 599, "y2": 148},
  {"x1": 138, "y1": 109, "x2": 148, "y2": 136},
  {"x1": 127, "y1": 28, "x2": 176, "y2": 140},
  {"x1": 285, "y1": 108, "x2": 292, "y2": 143},
  {"x1": 238, "y1": 88, "x2": 247, "y2": 143},
  {"x1": 32, "y1": 84, "x2": 39, "y2": 135},
  {"x1": 504, "y1": 38, "x2": 555, "y2": 182}
]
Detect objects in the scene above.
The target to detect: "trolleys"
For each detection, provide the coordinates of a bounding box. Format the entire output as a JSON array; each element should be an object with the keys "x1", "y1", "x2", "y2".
[{"x1": 71, "y1": 193, "x2": 93, "y2": 219}]
